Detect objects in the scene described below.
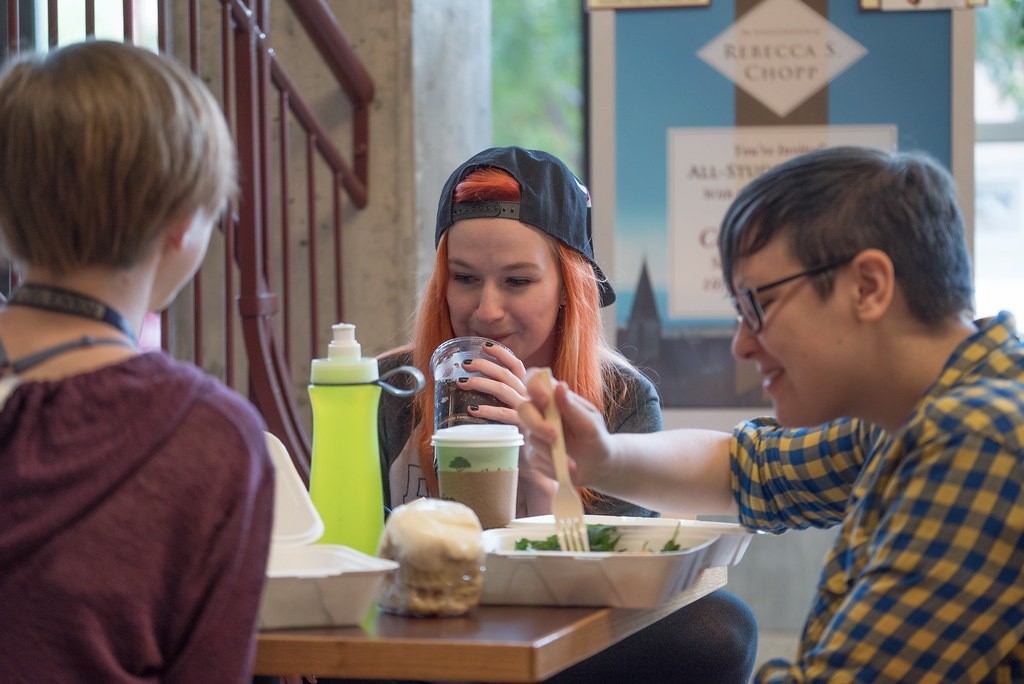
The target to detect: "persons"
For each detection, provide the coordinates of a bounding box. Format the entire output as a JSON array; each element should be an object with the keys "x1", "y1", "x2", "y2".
[
  {"x1": 373, "y1": 147, "x2": 758, "y2": 683},
  {"x1": 0, "y1": 40, "x2": 275, "y2": 684},
  {"x1": 519, "y1": 146, "x2": 1023, "y2": 684}
]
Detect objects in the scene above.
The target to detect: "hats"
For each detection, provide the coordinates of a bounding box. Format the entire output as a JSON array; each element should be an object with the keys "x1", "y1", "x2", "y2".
[{"x1": 434, "y1": 144, "x2": 621, "y2": 307}]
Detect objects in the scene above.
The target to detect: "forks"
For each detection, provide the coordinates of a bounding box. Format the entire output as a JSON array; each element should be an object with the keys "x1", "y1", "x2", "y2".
[{"x1": 533, "y1": 369, "x2": 591, "y2": 552}]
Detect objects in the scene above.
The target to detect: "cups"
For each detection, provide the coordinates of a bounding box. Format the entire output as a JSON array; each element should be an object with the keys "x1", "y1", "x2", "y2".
[
  {"x1": 430, "y1": 337, "x2": 519, "y2": 478},
  {"x1": 430, "y1": 423, "x2": 525, "y2": 530}
]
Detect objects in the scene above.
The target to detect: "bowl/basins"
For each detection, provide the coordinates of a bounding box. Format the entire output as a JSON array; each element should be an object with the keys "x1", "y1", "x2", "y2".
[
  {"x1": 262, "y1": 431, "x2": 324, "y2": 544},
  {"x1": 479, "y1": 514, "x2": 768, "y2": 611},
  {"x1": 258, "y1": 543, "x2": 399, "y2": 631}
]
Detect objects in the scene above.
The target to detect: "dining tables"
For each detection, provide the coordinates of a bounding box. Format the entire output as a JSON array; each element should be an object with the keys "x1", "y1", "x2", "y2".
[{"x1": 258, "y1": 566, "x2": 728, "y2": 684}]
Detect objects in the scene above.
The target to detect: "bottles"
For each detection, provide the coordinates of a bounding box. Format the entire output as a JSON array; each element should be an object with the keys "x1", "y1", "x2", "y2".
[{"x1": 306, "y1": 321, "x2": 425, "y2": 560}]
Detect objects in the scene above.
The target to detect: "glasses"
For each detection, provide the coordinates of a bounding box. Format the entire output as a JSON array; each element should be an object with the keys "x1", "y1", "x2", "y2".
[{"x1": 728, "y1": 253, "x2": 862, "y2": 335}]
